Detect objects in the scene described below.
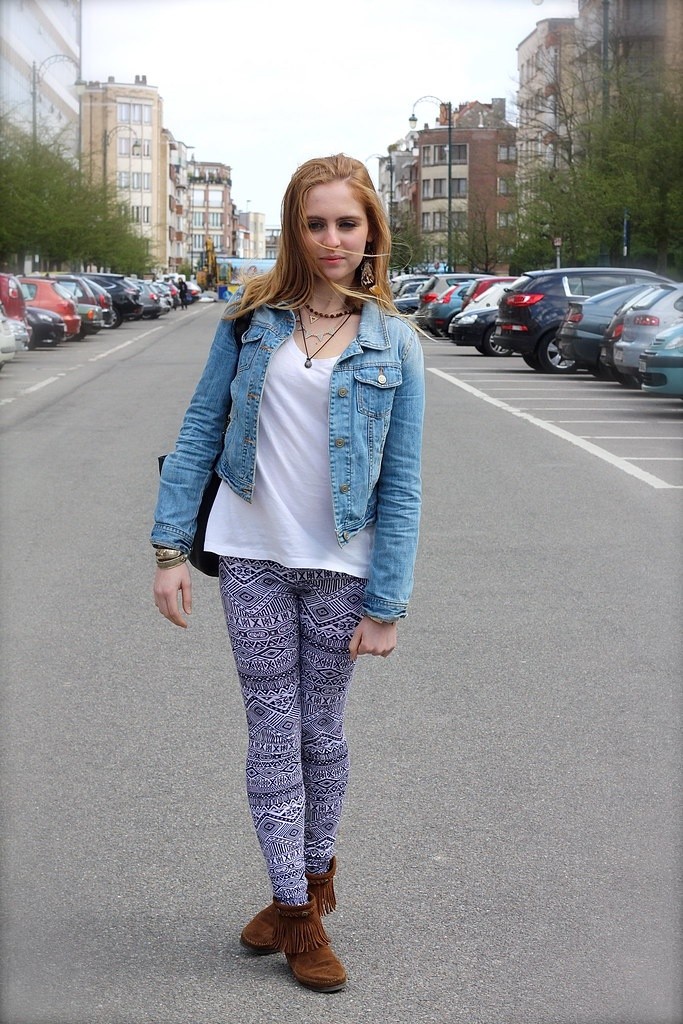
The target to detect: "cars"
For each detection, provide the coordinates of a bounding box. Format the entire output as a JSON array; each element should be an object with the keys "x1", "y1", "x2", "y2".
[
  {"x1": 448, "y1": 287, "x2": 523, "y2": 357},
  {"x1": 640, "y1": 323, "x2": 683, "y2": 398},
  {"x1": 557, "y1": 282, "x2": 652, "y2": 387},
  {"x1": 391, "y1": 273, "x2": 429, "y2": 313},
  {"x1": 25, "y1": 306, "x2": 67, "y2": 350}
]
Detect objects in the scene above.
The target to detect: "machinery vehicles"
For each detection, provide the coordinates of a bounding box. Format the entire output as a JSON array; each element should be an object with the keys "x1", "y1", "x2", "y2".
[{"x1": 197, "y1": 235, "x2": 222, "y2": 292}]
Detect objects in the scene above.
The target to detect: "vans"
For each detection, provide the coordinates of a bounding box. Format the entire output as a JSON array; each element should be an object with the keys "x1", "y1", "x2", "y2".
[{"x1": 464, "y1": 279, "x2": 523, "y2": 309}]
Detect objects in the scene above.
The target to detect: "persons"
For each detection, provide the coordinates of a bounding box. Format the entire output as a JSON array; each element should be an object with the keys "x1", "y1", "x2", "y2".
[
  {"x1": 171, "y1": 277, "x2": 188, "y2": 311},
  {"x1": 148, "y1": 152, "x2": 427, "y2": 993}
]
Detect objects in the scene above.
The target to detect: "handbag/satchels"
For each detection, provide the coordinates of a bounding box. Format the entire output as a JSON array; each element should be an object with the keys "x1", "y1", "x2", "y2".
[{"x1": 158, "y1": 293, "x2": 255, "y2": 577}]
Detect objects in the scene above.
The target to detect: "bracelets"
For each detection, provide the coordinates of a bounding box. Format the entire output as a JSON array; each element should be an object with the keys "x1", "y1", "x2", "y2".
[
  {"x1": 365, "y1": 614, "x2": 398, "y2": 626},
  {"x1": 155, "y1": 548, "x2": 188, "y2": 569}
]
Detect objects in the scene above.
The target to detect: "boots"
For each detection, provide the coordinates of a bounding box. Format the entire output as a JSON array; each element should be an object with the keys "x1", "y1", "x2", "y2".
[
  {"x1": 274, "y1": 889, "x2": 347, "y2": 992},
  {"x1": 239, "y1": 854, "x2": 336, "y2": 955}
]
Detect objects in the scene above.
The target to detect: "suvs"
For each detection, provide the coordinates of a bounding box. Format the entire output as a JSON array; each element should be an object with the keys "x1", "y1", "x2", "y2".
[
  {"x1": 415, "y1": 273, "x2": 499, "y2": 338},
  {"x1": 612, "y1": 283, "x2": 683, "y2": 380},
  {"x1": 11, "y1": 278, "x2": 79, "y2": 342},
  {"x1": 494, "y1": 268, "x2": 678, "y2": 374},
  {"x1": 0, "y1": 273, "x2": 33, "y2": 374},
  {"x1": 19, "y1": 272, "x2": 201, "y2": 348}
]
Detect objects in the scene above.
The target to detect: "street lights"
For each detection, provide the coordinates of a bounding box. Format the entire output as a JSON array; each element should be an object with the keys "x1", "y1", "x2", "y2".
[
  {"x1": 104, "y1": 126, "x2": 140, "y2": 206},
  {"x1": 409, "y1": 95, "x2": 452, "y2": 273},
  {"x1": 31, "y1": 55, "x2": 87, "y2": 151}
]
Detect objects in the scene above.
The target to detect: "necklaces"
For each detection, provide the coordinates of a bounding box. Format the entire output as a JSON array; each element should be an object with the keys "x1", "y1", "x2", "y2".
[{"x1": 295, "y1": 296, "x2": 354, "y2": 369}]
